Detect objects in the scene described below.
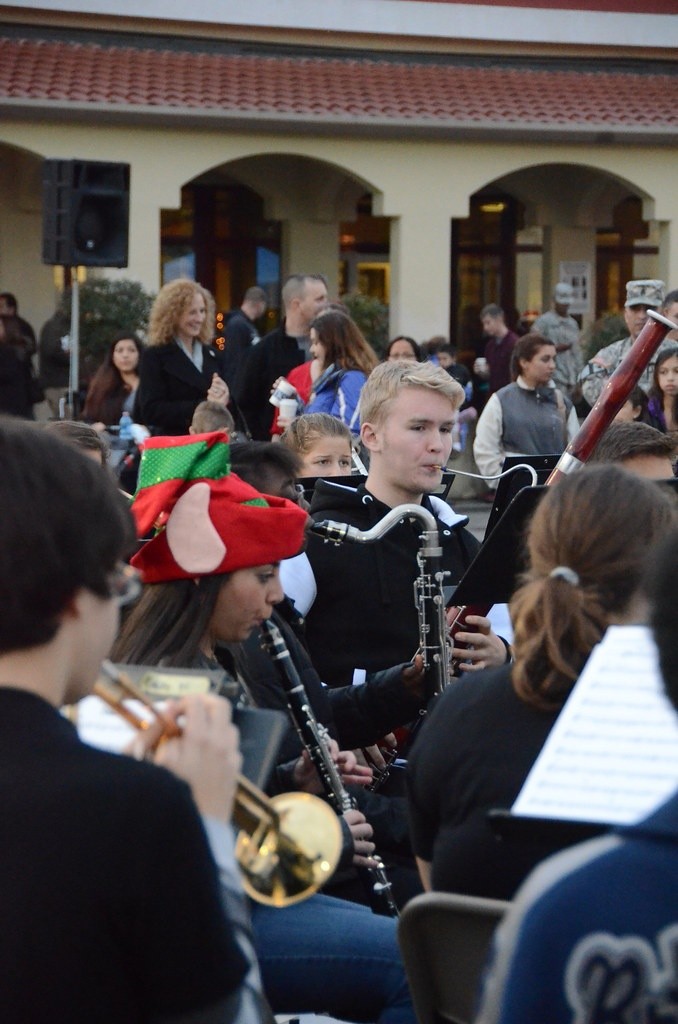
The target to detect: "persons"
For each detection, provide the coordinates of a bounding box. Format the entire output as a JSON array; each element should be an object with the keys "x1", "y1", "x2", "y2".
[
  {"x1": 111, "y1": 431, "x2": 417, "y2": 1024},
  {"x1": 0, "y1": 419, "x2": 264, "y2": 1024},
  {"x1": 482, "y1": 520, "x2": 678, "y2": 1024},
  {"x1": 405, "y1": 466, "x2": 678, "y2": 900},
  {"x1": 0, "y1": 274, "x2": 678, "y2": 918}
]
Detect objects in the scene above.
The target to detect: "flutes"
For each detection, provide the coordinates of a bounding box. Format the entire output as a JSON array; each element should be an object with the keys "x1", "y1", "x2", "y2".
[{"x1": 259, "y1": 618, "x2": 401, "y2": 918}]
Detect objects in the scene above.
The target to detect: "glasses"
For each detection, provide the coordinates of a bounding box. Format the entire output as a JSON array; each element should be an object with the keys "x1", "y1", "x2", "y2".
[
  {"x1": 386, "y1": 353, "x2": 417, "y2": 360},
  {"x1": 112, "y1": 559, "x2": 140, "y2": 607},
  {"x1": 114, "y1": 345, "x2": 141, "y2": 355}
]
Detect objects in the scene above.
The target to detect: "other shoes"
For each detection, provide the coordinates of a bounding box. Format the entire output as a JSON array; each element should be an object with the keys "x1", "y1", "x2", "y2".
[
  {"x1": 484, "y1": 495, "x2": 496, "y2": 502},
  {"x1": 450, "y1": 448, "x2": 460, "y2": 459},
  {"x1": 475, "y1": 490, "x2": 491, "y2": 498}
]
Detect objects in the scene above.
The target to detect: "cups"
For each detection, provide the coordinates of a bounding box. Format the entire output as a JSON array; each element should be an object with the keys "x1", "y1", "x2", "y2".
[
  {"x1": 475, "y1": 357, "x2": 486, "y2": 373},
  {"x1": 279, "y1": 399, "x2": 299, "y2": 426},
  {"x1": 268, "y1": 379, "x2": 296, "y2": 408}
]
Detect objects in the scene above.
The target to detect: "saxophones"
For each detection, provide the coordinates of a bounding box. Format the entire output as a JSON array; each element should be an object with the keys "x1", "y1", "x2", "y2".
[{"x1": 310, "y1": 502, "x2": 451, "y2": 715}]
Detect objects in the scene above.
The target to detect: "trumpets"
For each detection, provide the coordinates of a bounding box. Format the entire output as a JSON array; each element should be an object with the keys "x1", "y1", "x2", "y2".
[{"x1": 92, "y1": 656, "x2": 343, "y2": 907}]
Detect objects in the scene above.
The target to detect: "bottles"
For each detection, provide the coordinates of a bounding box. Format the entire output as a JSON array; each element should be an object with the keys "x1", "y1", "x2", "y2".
[{"x1": 119, "y1": 411, "x2": 134, "y2": 440}]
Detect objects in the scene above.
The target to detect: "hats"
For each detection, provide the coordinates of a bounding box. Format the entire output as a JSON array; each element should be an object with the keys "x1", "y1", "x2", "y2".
[
  {"x1": 624, "y1": 279, "x2": 666, "y2": 307},
  {"x1": 555, "y1": 282, "x2": 575, "y2": 305},
  {"x1": 129, "y1": 431, "x2": 308, "y2": 584}
]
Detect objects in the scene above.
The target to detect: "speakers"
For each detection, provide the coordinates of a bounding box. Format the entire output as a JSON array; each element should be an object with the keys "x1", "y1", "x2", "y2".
[{"x1": 41, "y1": 158, "x2": 131, "y2": 269}]
